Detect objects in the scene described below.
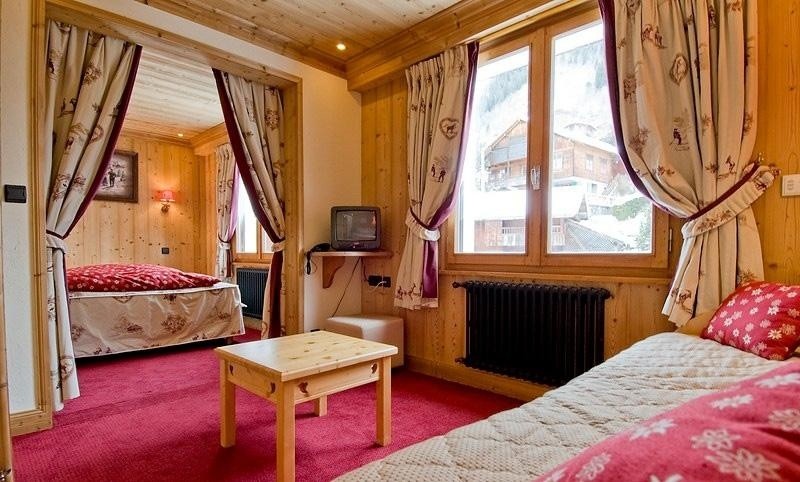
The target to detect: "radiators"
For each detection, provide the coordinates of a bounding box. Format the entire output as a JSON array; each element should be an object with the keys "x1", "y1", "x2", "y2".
[
  {"x1": 235, "y1": 267, "x2": 269, "y2": 320},
  {"x1": 449, "y1": 277, "x2": 612, "y2": 395}
]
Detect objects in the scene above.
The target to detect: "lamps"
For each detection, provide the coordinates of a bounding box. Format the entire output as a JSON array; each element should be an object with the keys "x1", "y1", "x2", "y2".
[{"x1": 156, "y1": 188, "x2": 176, "y2": 216}]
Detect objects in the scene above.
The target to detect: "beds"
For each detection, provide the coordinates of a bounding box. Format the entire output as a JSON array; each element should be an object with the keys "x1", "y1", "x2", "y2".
[
  {"x1": 65, "y1": 262, "x2": 247, "y2": 362},
  {"x1": 324, "y1": 329, "x2": 799, "y2": 482}
]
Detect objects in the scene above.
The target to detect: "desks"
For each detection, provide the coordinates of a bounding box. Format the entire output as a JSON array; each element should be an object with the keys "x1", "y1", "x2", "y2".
[{"x1": 213, "y1": 328, "x2": 395, "y2": 482}]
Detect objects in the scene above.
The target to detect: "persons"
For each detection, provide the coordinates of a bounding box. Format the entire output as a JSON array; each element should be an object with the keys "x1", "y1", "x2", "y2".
[
  {"x1": 115, "y1": 165, "x2": 129, "y2": 182},
  {"x1": 108, "y1": 169, "x2": 117, "y2": 187}
]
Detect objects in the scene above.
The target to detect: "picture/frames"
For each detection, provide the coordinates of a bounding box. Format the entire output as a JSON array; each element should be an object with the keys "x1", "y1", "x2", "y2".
[{"x1": 92, "y1": 148, "x2": 139, "y2": 203}]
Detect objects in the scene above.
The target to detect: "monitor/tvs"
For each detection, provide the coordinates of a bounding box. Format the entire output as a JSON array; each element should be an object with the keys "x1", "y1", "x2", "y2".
[{"x1": 331, "y1": 206, "x2": 381, "y2": 250}]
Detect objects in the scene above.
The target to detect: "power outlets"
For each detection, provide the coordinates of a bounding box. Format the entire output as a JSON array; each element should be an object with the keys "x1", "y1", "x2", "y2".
[
  {"x1": 161, "y1": 247, "x2": 169, "y2": 254},
  {"x1": 781, "y1": 174, "x2": 800, "y2": 197},
  {"x1": 366, "y1": 275, "x2": 392, "y2": 287}
]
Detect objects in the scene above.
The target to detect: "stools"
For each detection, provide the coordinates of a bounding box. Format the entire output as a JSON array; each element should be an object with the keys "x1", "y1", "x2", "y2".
[{"x1": 325, "y1": 312, "x2": 405, "y2": 370}]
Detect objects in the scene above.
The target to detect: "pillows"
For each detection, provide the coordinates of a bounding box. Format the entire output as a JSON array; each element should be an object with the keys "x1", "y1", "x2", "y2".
[{"x1": 699, "y1": 278, "x2": 799, "y2": 361}]
